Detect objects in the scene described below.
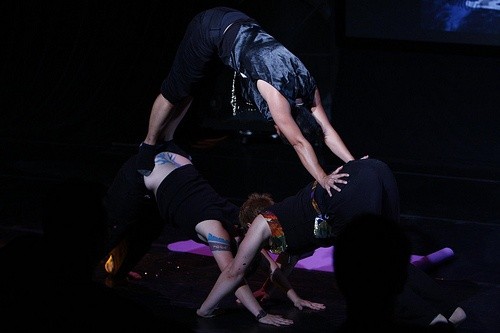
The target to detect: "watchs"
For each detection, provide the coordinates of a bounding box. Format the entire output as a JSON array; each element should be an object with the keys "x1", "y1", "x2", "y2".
[{"x1": 256, "y1": 310, "x2": 267, "y2": 319}]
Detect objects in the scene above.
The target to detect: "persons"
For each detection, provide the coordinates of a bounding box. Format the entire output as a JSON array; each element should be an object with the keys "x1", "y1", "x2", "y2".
[
  {"x1": 197, "y1": 158, "x2": 479, "y2": 333},
  {"x1": 134, "y1": 6, "x2": 370, "y2": 197},
  {"x1": 101, "y1": 148, "x2": 327, "y2": 327}
]
